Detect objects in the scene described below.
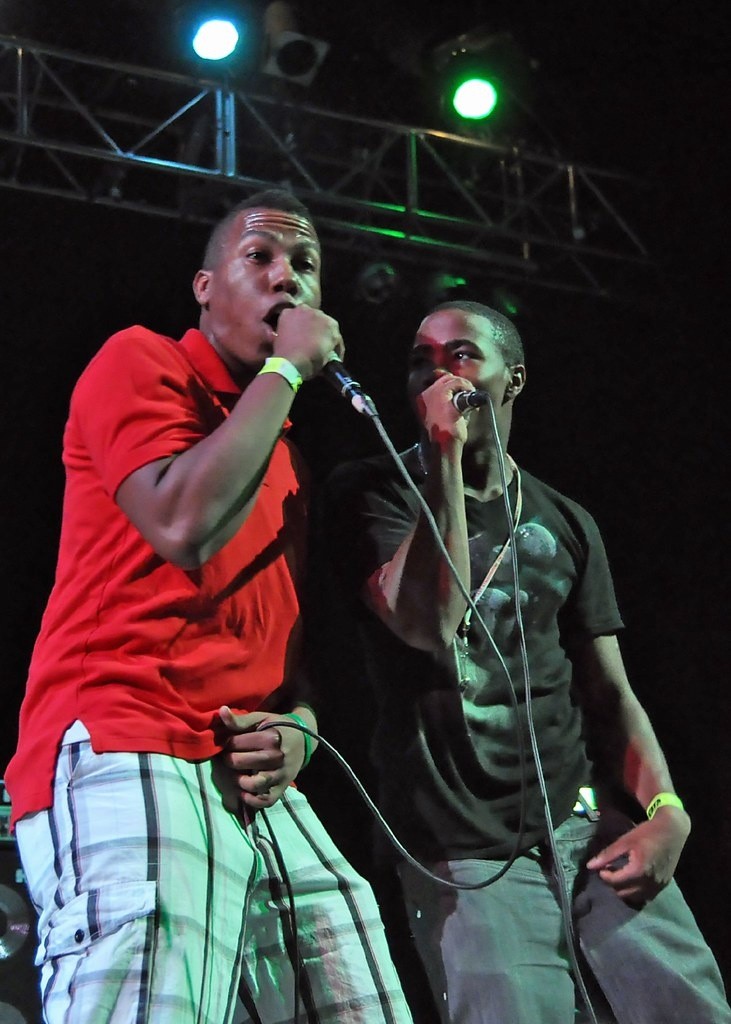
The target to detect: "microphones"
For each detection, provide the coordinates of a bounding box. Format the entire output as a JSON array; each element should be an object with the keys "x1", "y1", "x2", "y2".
[
  {"x1": 319, "y1": 351, "x2": 380, "y2": 418},
  {"x1": 452, "y1": 390, "x2": 491, "y2": 414}
]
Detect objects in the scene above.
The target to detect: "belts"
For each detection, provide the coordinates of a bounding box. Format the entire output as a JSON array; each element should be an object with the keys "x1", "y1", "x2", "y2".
[{"x1": 571, "y1": 786, "x2": 613, "y2": 812}]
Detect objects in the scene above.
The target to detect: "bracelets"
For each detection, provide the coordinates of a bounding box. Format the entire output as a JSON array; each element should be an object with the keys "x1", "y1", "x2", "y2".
[
  {"x1": 646, "y1": 792, "x2": 683, "y2": 821},
  {"x1": 256, "y1": 355, "x2": 303, "y2": 393},
  {"x1": 283, "y1": 712, "x2": 311, "y2": 770}
]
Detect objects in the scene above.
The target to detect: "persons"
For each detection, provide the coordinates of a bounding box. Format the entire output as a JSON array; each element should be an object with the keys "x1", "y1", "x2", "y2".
[
  {"x1": 5, "y1": 189, "x2": 412, "y2": 1024},
  {"x1": 319, "y1": 301, "x2": 731, "y2": 1023}
]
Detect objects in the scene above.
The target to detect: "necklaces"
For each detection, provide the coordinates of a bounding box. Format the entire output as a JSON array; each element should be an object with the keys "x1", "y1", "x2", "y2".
[{"x1": 417, "y1": 444, "x2": 428, "y2": 474}]
